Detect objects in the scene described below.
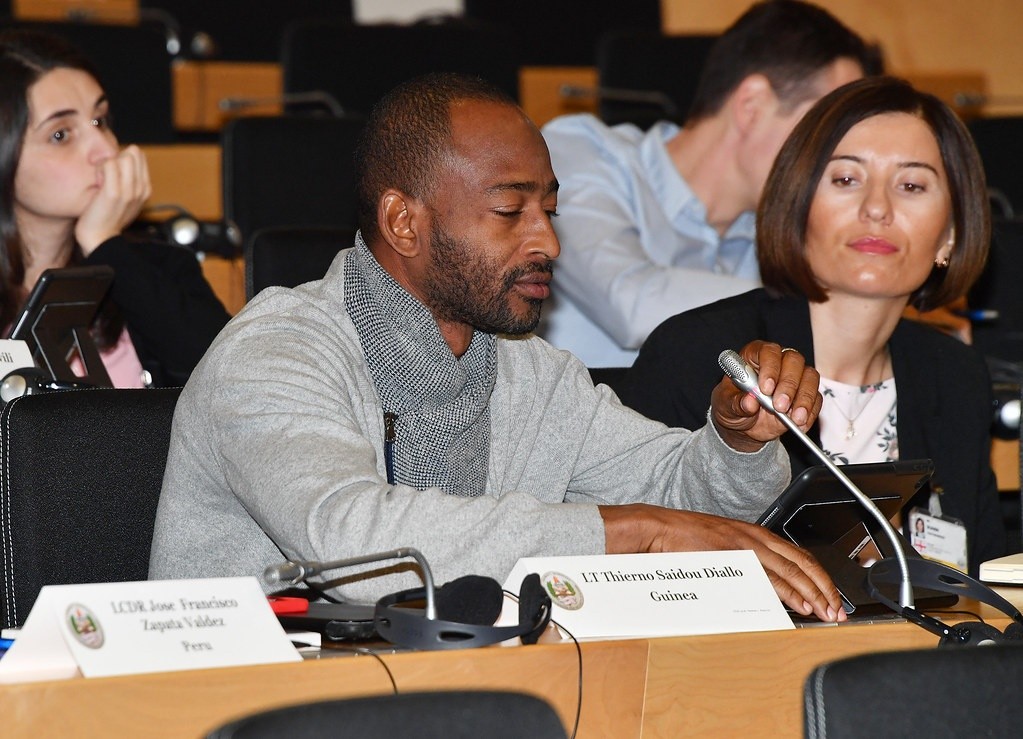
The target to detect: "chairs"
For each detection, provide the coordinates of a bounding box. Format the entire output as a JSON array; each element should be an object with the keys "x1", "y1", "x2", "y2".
[
  {"x1": 464, "y1": 0, "x2": 718, "y2": 133},
  {"x1": 222, "y1": 21, "x2": 523, "y2": 304},
  {"x1": 0, "y1": 386, "x2": 185, "y2": 634},
  {"x1": 16, "y1": 20, "x2": 172, "y2": 146}
]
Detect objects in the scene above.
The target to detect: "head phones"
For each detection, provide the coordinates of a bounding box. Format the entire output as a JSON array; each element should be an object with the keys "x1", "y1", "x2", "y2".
[
  {"x1": 373, "y1": 573, "x2": 552, "y2": 650},
  {"x1": 862, "y1": 556, "x2": 1023, "y2": 649},
  {"x1": 0, "y1": 367, "x2": 99, "y2": 409}
]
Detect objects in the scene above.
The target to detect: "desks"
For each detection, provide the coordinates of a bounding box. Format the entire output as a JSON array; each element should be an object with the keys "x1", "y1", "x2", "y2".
[
  {"x1": 659, "y1": 0, "x2": 1023, "y2": 125},
  {"x1": 140, "y1": 60, "x2": 282, "y2": 223},
  {"x1": 0, "y1": 583, "x2": 1023, "y2": 739}
]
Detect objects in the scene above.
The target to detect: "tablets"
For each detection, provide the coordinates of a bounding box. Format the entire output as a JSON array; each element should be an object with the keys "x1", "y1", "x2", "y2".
[
  {"x1": 6, "y1": 265, "x2": 115, "y2": 363},
  {"x1": 753, "y1": 458, "x2": 936, "y2": 560}
]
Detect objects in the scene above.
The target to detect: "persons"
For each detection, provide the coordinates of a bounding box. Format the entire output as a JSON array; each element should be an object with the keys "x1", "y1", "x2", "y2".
[
  {"x1": 0, "y1": 17, "x2": 235, "y2": 392},
  {"x1": 147, "y1": 69, "x2": 848, "y2": 625},
  {"x1": 623, "y1": 74, "x2": 1005, "y2": 595},
  {"x1": 536, "y1": 0, "x2": 880, "y2": 368},
  {"x1": 915, "y1": 519, "x2": 925, "y2": 537}
]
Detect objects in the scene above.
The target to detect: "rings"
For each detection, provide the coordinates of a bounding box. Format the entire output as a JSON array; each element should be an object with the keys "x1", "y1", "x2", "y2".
[{"x1": 782, "y1": 348, "x2": 799, "y2": 354}]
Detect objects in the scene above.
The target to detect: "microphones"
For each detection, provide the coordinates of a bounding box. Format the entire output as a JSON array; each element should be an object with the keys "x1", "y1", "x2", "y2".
[
  {"x1": 219, "y1": 93, "x2": 346, "y2": 119},
  {"x1": 718, "y1": 349, "x2": 914, "y2": 618},
  {"x1": 560, "y1": 84, "x2": 677, "y2": 115},
  {"x1": 263, "y1": 548, "x2": 438, "y2": 621}
]
